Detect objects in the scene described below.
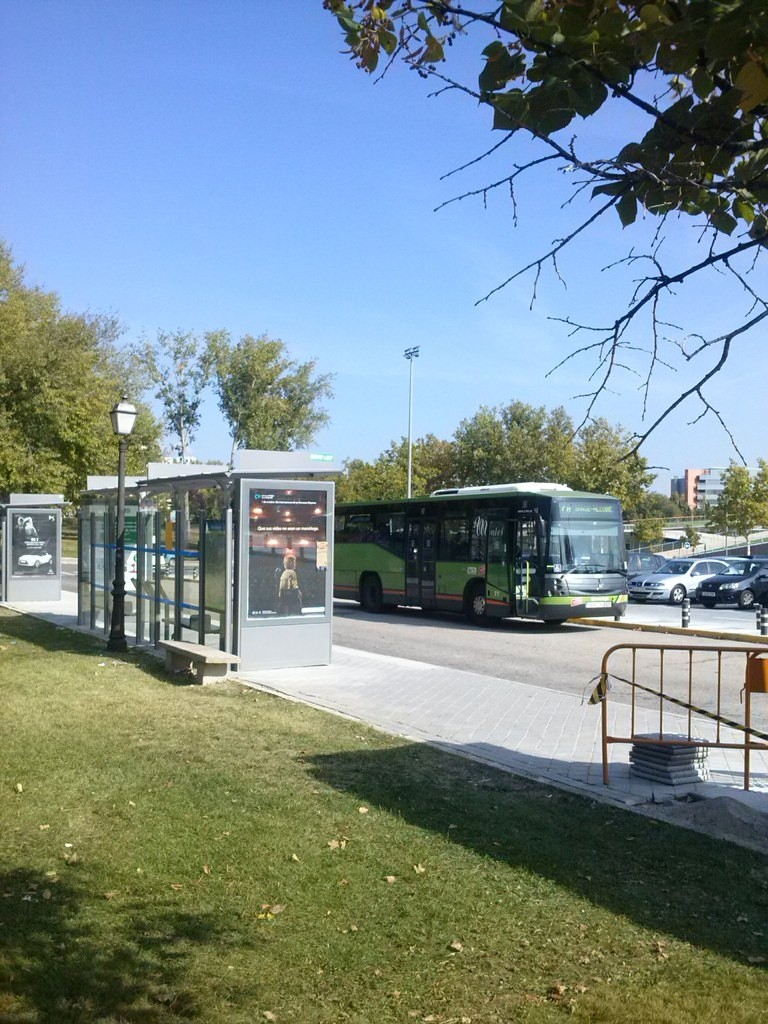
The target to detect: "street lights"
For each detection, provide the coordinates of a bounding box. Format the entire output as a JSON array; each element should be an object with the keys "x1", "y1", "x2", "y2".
[
  {"x1": 403, "y1": 344, "x2": 421, "y2": 495},
  {"x1": 107, "y1": 395, "x2": 139, "y2": 650}
]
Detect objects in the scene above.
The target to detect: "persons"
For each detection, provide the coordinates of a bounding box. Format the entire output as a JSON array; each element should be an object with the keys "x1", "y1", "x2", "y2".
[{"x1": 278, "y1": 555, "x2": 302, "y2": 599}]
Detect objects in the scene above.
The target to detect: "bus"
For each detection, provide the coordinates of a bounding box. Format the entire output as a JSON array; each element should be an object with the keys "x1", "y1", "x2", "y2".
[{"x1": 334, "y1": 482, "x2": 630, "y2": 625}]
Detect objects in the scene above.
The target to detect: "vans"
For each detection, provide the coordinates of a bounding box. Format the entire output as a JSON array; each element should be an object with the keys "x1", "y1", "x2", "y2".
[{"x1": 124, "y1": 535, "x2": 166, "y2": 579}]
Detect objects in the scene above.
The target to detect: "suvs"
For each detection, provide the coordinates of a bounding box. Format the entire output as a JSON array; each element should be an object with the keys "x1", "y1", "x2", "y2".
[{"x1": 587, "y1": 553, "x2": 668, "y2": 582}]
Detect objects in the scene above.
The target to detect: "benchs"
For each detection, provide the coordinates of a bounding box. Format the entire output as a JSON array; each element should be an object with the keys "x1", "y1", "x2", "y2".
[
  {"x1": 162, "y1": 618, "x2": 220, "y2": 635},
  {"x1": 93, "y1": 603, "x2": 136, "y2": 616},
  {"x1": 156, "y1": 639, "x2": 241, "y2": 686}
]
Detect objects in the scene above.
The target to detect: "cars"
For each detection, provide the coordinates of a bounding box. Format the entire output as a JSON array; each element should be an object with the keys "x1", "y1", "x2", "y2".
[
  {"x1": 19, "y1": 549, "x2": 53, "y2": 569},
  {"x1": 628, "y1": 558, "x2": 740, "y2": 605},
  {"x1": 705, "y1": 555, "x2": 758, "y2": 575},
  {"x1": 161, "y1": 546, "x2": 176, "y2": 566},
  {"x1": 696, "y1": 558, "x2": 767, "y2": 610}
]
